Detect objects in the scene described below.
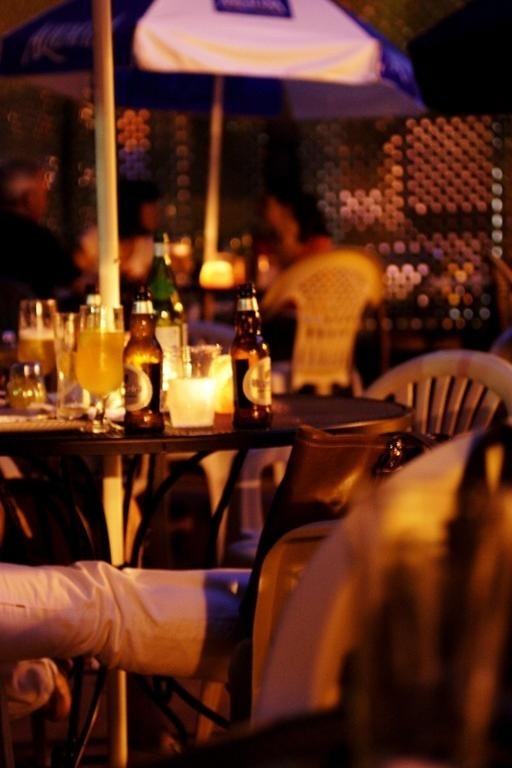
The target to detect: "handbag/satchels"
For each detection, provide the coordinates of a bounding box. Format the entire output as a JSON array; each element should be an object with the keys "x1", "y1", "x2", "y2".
[{"x1": 238, "y1": 424, "x2": 455, "y2": 644}]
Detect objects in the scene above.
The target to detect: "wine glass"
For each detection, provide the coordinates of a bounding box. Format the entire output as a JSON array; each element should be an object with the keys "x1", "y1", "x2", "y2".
[{"x1": 7, "y1": 295, "x2": 125, "y2": 424}]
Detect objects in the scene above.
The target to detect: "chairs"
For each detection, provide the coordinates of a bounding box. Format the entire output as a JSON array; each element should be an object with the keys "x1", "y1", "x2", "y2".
[{"x1": 217, "y1": 351, "x2": 511, "y2": 767}]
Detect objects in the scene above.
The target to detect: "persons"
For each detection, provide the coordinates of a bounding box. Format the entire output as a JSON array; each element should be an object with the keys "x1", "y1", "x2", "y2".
[
  {"x1": 254, "y1": 191, "x2": 338, "y2": 358},
  {"x1": 77, "y1": 176, "x2": 165, "y2": 288},
  {"x1": 0, "y1": 160, "x2": 95, "y2": 341}
]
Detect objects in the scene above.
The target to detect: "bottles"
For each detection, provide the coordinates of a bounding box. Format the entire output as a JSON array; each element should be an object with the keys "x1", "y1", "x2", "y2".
[{"x1": 125, "y1": 231, "x2": 273, "y2": 433}]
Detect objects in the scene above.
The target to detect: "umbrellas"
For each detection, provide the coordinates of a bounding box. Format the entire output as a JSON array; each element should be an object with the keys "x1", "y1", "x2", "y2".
[{"x1": 0, "y1": 0, "x2": 425, "y2": 260}]
